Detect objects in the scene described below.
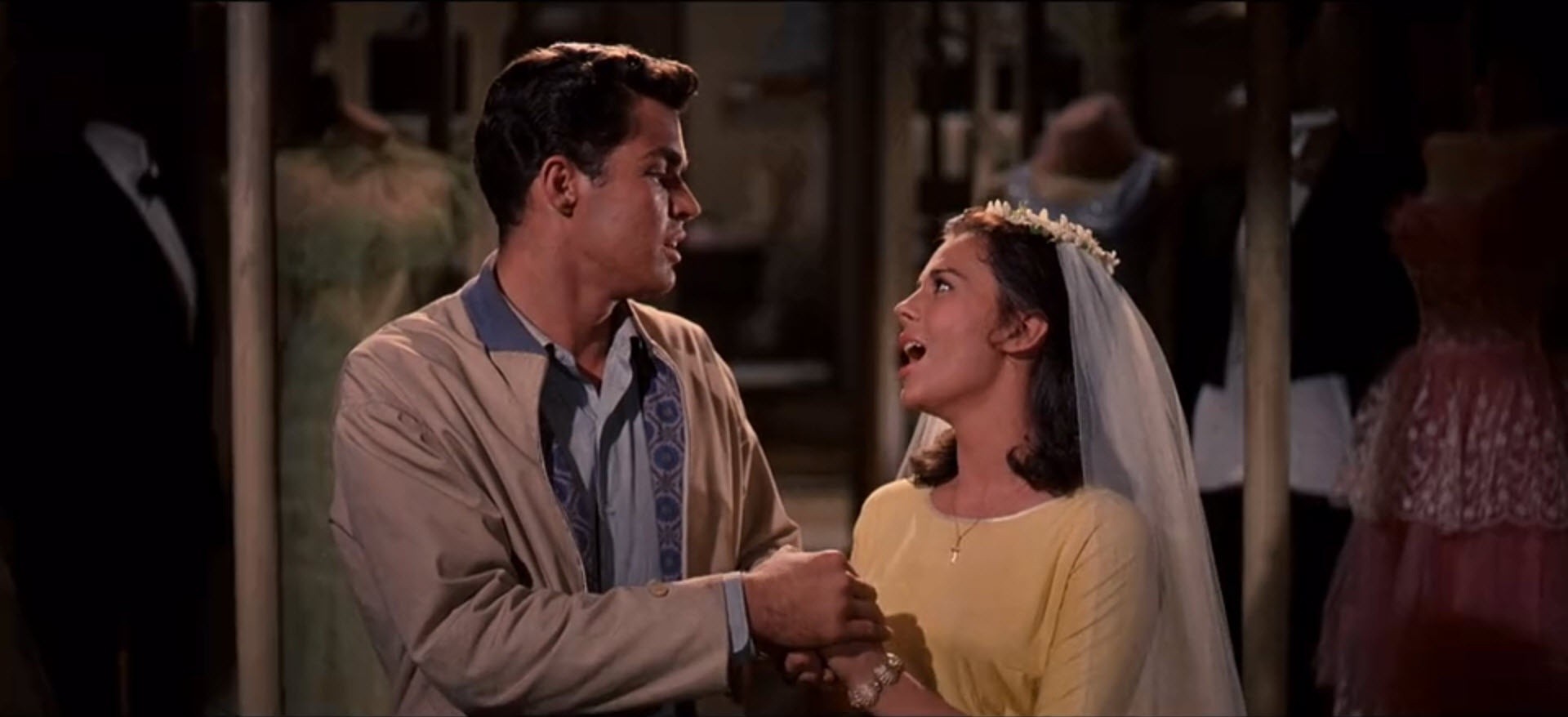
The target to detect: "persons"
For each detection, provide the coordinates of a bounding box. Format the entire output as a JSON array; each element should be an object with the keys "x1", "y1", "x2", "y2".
[
  {"x1": 1, "y1": 1, "x2": 490, "y2": 717},
  {"x1": 327, "y1": 40, "x2": 1248, "y2": 717},
  {"x1": 1033, "y1": 4, "x2": 1568, "y2": 717}
]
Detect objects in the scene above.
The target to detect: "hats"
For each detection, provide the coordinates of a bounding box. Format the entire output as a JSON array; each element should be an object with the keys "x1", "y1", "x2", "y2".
[{"x1": 1003, "y1": 93, "x2": 1155, "y2": 237}]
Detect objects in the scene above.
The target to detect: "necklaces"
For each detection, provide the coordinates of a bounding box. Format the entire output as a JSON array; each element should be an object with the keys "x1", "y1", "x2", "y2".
[{"x1": 950, "y1": 478, "x2": 1029, "y2": 564}]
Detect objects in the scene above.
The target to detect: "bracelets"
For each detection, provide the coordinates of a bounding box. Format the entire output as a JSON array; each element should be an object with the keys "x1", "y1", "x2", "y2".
[{"x1": 850, "y1": 652, "x2": 905, "y2": 709}]
